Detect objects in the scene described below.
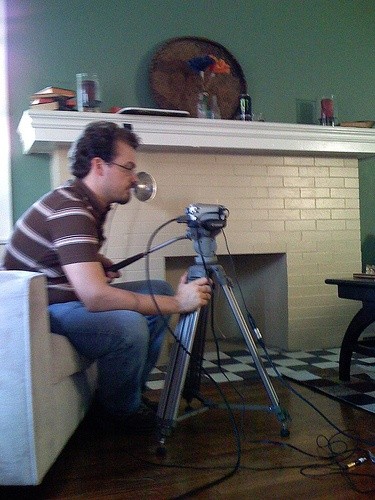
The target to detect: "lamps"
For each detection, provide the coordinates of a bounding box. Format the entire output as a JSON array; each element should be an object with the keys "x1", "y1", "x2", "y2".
[{"x1": 135, "y1": 171, "x2": 157, "y2": 203}]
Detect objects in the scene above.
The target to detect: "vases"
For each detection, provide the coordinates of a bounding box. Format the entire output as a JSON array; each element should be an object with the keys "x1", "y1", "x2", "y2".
[{"x1": 198, "y1": 89, "x2": 221, "y2": 119}]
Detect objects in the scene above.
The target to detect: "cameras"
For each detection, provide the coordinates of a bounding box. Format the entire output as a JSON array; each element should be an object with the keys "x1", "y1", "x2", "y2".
[{"x1": 186, "y1": 203, "x2": 228, "y2": 229}]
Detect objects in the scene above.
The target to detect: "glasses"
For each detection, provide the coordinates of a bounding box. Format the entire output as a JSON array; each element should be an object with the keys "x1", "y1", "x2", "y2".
[{"x1": 106, "y1": 160, "x2": 137, "y2": 176}]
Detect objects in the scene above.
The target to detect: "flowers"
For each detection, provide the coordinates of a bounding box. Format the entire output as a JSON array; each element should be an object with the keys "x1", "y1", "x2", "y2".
[{"x1": 188, "y1": 54, "x2": 230, "y2": 89}]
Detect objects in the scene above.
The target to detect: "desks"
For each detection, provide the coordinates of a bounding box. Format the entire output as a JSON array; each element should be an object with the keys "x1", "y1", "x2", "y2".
[{"x1": 325, "y1": 276, "x2": 374, "y2": 380}]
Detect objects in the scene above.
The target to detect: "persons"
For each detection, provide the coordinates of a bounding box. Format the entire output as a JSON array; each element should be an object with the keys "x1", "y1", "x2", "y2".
[{"x1": 4, "y1": 120, "x2": 211, "y2": 441}]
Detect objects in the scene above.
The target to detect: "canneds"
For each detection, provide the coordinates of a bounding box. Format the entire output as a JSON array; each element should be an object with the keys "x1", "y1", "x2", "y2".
[{"x1": 238, "y1": 93, "x2": 252, "y2": 121}]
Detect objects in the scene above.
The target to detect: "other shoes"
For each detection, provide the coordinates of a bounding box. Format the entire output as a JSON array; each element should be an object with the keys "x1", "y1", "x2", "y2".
[{"x1": 88, "y1": 396, "x2": 159, "y2": 439}]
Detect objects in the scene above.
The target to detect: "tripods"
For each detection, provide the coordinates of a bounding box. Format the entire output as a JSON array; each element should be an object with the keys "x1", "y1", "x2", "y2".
[{"x1": 105, "y1": 230, "x2": 291, "y2": 457}]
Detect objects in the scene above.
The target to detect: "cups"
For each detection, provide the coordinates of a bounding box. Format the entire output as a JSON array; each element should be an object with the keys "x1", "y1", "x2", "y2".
[
  {"x1": 75, "y1": 72, "x2": 101, "y2": 113},
  {"x1": 320, "y1": 95, "x2": 337, "y2": 127}
]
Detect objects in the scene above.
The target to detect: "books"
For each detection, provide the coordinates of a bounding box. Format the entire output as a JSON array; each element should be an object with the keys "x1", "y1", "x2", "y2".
[{"x1": 28, "y1": 85, "x2": 79, "y2": 111}]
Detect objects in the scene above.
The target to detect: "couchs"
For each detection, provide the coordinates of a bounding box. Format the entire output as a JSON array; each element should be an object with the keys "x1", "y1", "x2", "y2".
[{"x1": 1, "y1": 245, "x2": 97, "y2": 487}]
